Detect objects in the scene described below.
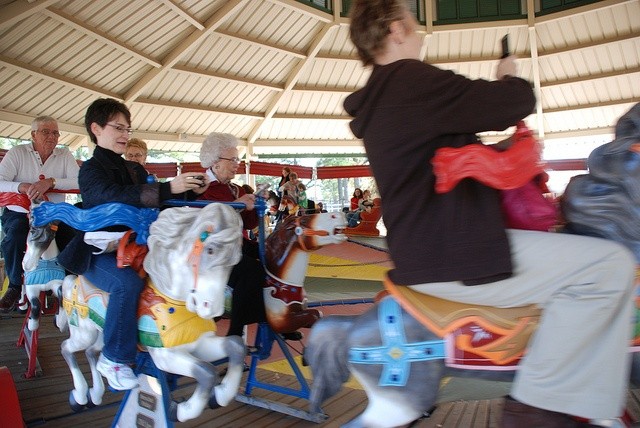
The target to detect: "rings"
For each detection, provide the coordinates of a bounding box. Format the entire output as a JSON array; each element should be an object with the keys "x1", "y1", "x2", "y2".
[{"x1": 36, "y1": 192, "x2": 40, "y2": 194}]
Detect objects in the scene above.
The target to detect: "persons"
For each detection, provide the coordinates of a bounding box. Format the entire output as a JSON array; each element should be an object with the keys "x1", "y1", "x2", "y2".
[
  {"x1": 350, "y1": 187, "x2": 363, "y2": 211},
  {"x1": 198, "y1": 133, "x2": 303, "y2": 374},
  {"x1": 276, "y1": 166, "x2": 291, "y2": 197},
  {"x1": 339, "y1": 0, "x2": 636, "y2": 426},
  {"x1": 318, "y1": 202, "x2": 327, "y2": 213},
  {"x1": 1, "y1": 116, "x2": 81, "y2": 313},
  {"x1": 55, "y1": 98, "x2": 209, "y2": 391},
  {"x1": 297, "y1": 183, "x2": 308, "y2": 209},
  {"x1": 346, "y1": 190, "x2": 373, "y2": 228},
  {"x1": 124, "y1": 138, "x2": 149, "y2": 168},
  {"x1": 277, "y1": 172, "x2": 301, "y2": 202}
]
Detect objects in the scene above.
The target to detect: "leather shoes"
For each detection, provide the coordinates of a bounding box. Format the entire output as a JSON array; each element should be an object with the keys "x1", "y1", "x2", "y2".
[{"x1": 497, "y1": 395, "x2": 608, "y2": 428}]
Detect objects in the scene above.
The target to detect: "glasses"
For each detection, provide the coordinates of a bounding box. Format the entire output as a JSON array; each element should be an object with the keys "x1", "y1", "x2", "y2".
[
  {"x1": 34, "y1": 128, "x2": 60, "y2": 137},
  {"x1": 219, "y1": 157, "x2": 241, "y2": 163},
  {"x1": 106, "y1": 124, "x2": 133, "y2": 135},
  {"x1": 126, "y1": 153, "x2": 146, "y2": 159}
]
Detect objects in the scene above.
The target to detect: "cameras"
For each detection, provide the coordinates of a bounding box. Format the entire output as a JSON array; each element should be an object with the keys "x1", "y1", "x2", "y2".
[{"x1": 193, "y1": 175, "x2": 205, "y2": 187}]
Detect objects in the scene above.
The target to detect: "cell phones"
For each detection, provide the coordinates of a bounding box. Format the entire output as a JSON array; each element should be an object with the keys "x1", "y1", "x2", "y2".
[{"x1": 499, "y1": 34, "x2": 511, "y2": 58}]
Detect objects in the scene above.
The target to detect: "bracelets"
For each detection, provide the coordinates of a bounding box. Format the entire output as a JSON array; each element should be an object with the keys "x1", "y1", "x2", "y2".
[{"x1": 50, "y1": 178, "x2": 55, "y2": 187}]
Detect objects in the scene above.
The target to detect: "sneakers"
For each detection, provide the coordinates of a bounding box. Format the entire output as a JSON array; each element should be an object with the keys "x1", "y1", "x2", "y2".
[
  {"x1": 96, "y1": 351, "x2": 140, "y2": 391},
  {"x1": 0, "y1": 287, "x2": 21, "y2": 310}
]
